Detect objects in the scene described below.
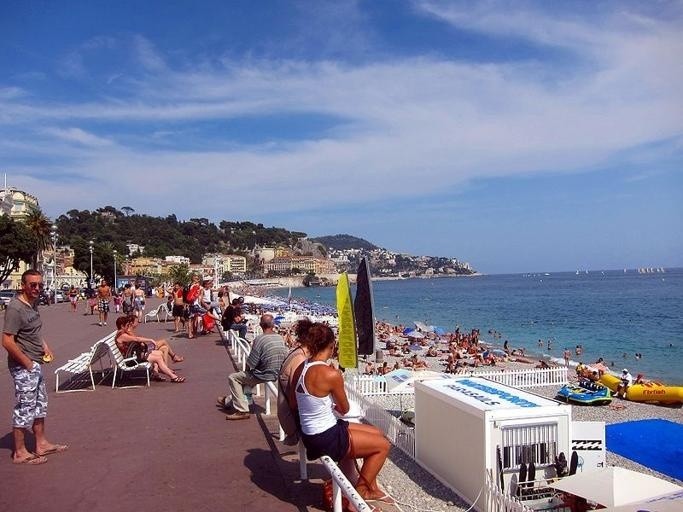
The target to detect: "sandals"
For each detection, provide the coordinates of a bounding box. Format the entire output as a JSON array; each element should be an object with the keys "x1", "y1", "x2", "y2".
[{"x1": 151, "y1": 372, "x2": 185, "y2": 382}]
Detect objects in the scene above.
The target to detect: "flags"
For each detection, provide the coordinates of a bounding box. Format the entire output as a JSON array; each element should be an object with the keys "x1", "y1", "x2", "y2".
[{"x1": 352, "y1": 255, "x2": 375, "y2": 356}]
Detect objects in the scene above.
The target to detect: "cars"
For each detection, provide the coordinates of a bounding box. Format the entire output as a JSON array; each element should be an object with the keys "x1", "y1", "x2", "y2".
[{"x1": 0, "y1": 287, "x2": 97, "y2": 309}]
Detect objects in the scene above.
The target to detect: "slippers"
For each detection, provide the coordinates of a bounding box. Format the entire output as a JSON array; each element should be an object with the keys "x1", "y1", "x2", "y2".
[
  {"x1": 365, "y1": 495, "x2": 393, "y2": 504},
  {"x1": 13, "y1": 445, "x2": 68, "y2": 464}
]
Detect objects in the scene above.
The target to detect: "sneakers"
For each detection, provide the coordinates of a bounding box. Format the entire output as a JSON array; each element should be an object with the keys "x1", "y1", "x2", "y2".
[
  {"x1": 218, "y1": 397, "x2": 226, "y2": 404},
  {"x1": 226, "y1": 411, "x2": 250, "y2": 420}
]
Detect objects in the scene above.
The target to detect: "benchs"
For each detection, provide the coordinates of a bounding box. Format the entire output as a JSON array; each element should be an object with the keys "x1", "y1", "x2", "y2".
[
  {"x1": 143, "y1": 303, "x2": 175, "y2": 324},
  {"x1": 54, "y1": 330, "x2": 152, "y2": 394}
]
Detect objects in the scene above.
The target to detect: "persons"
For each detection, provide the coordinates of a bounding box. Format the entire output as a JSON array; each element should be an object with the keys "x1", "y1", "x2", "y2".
[
  {"x1": 69, "y1": 285, "x2": 79, "y2": 312},
  {"x1": 216, "y1": 314, "x2": 285, "y2": 420},
  {"x1": 165, "y1": 275, "x2": 248, "y2": 342},
  {"x1": 275, "y1": 314, "x2": 359, "y2": 489},
  {"x1": 364, "y1": 324, "x2": 642, "y2": 397},
  {"x1": 85, "y1": 281, "x2": 146, "y2": 325},
  {"x1": 44, "y1": 288, "x2": 53, "y2": 306},
  {"x1": 114, "y1": 315, "x2": 186, "y2": 383},
  {"x1": 287, "y1": 322, "x2": 393, "y2": 512},
  {"x1": 0, "y1": 269, "x2": 68, "y2": 465}
]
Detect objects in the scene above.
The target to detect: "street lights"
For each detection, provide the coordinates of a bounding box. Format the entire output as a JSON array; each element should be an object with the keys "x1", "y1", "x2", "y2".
[
  {"x1": 49, "y1": 221, "x2": 62, "y2": 303},
  {"x1": 87, "y1": 237, "x2": 96, "y2": 289},
  {"x1": 124, "y1": 253, "x2": 133, "y2": 285},
  {"x1": 110, "y1": 249, "x2": 118, "y2": 294}
]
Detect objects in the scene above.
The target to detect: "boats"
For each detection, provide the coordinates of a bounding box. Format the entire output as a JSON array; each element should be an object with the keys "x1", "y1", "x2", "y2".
[
  {"x1": 557, "y1": 380, "x2": 613, "y2": 405},
  {"x1": 575, "y1": 363, "x2": 682, "y2": 408}
]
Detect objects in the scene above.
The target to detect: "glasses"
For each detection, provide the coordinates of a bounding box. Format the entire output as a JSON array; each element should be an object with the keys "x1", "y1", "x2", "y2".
[{"x1": 30, "y1": 282, "x2": 43, "y2": 288}]
